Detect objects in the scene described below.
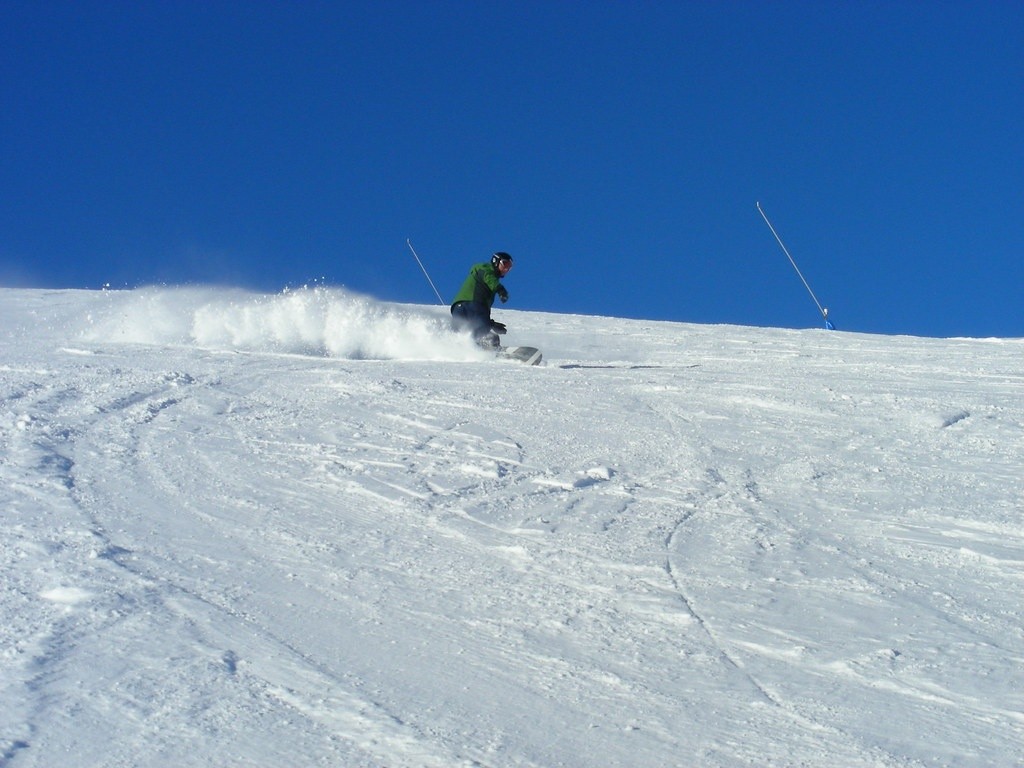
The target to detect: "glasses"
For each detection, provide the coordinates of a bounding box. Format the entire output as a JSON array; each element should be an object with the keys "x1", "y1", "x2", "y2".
[{"x1": 500, "y1": 260, "x2": 511, "y2": 271}]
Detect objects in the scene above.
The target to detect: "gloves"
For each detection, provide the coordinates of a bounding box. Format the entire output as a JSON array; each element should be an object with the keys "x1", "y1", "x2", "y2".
[
  {"x1": 496, "y1": 285, "x2": 508, "y2": 303},
  {"x1": 490, "y1": 319, "x2": 506, "y2": 334}
]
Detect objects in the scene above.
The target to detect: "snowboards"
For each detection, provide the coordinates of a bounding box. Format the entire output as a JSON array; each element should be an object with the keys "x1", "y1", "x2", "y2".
[{"x1": 477, "y1": 345, "x2": 544, "y2": 367}]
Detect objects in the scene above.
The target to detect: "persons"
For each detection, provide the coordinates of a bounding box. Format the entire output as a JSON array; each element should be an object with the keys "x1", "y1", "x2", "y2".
[{"x1": 451, "y1": 252, "x2": 512, "y2": 349}]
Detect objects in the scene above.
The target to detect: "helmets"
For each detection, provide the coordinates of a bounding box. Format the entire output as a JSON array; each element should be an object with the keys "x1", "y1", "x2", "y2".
[{"x1": 491, "y1": 252, "x2": 513, "y2": 278}]
